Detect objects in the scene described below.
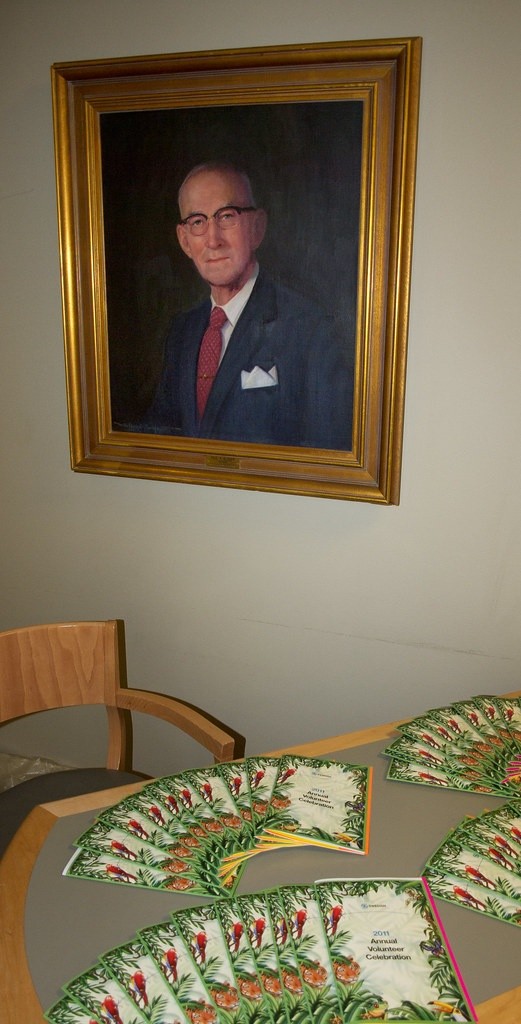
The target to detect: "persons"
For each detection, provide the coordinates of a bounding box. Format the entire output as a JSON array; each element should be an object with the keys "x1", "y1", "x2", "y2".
[{"x1": 143, "y1": 162, "x2": 351, "y2": 450}]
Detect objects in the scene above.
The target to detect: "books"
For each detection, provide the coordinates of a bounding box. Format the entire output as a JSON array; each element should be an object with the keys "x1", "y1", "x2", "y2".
[
  {"x1": 61, "y1": 755, "x2": 372, "y2": 898},
  {"x1": 382, "y1": 694, "x2": 521, "y2": 801},
  {"x1": 42, "y1": 876, "x2": 479, "y2": 1023},
  {"x1": 416, "y1": 799, "x2": 521, "y2": 928}
]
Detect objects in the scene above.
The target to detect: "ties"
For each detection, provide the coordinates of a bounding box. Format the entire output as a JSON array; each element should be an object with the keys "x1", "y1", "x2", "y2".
[{"x1": 195, "y1": 307, "x2": 227, "y2": 431}]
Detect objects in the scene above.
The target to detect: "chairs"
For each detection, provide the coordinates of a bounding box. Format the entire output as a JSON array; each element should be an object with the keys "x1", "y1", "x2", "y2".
[{"x1": 0, "y1": 619, "x2": 235, "y2": 857}]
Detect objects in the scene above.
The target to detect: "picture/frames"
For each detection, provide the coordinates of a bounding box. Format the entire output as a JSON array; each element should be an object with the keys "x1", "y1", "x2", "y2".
[{"x1": 50, "y1": 35, "x2": 424, "y2": 506}]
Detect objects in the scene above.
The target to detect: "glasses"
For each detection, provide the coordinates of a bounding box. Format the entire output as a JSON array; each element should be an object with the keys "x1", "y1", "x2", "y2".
[{"x1": 178, "y1": 205, "x2": 258, "y2": 237}]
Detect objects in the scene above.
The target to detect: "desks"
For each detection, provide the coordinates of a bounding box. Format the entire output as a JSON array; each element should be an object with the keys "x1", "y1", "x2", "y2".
[{"x1": 0, "y1": 689, "x2": 521, "y2": 1024}]
